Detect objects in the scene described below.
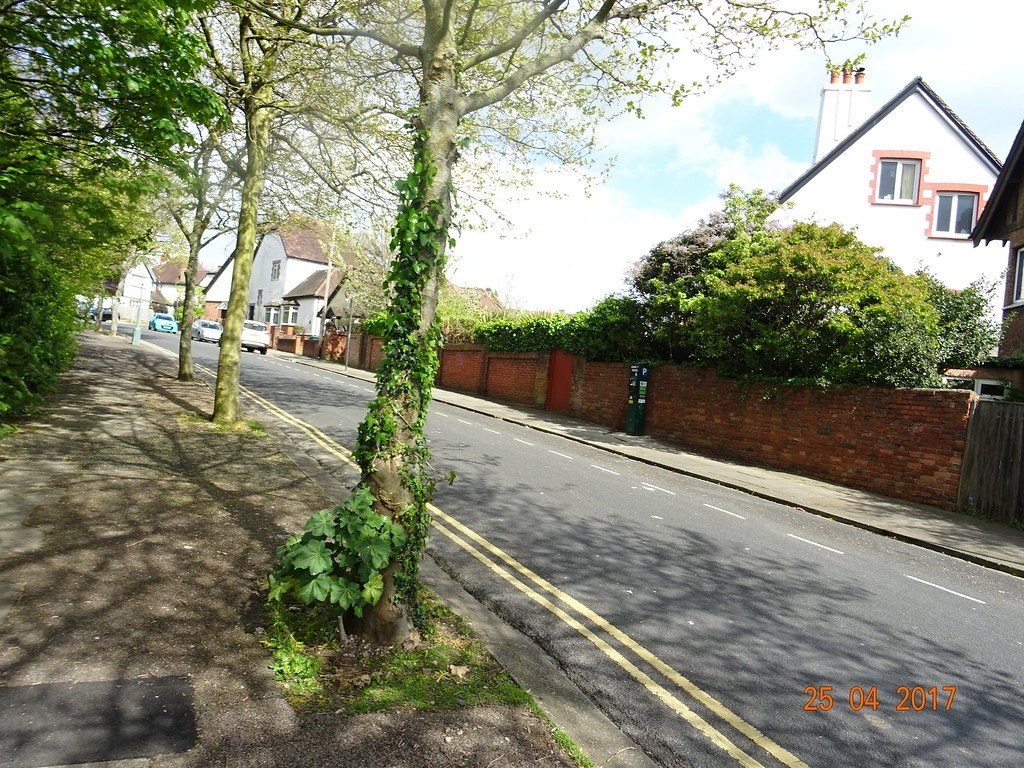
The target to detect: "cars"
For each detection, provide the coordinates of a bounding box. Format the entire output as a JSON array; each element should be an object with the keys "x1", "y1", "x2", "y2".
[
  {"x1": 89, "y1": 306, "x2": 121, "y2": 322},
  {"x1": 148, "y1": 312, "x2": 180, "y2": 335},
  {"x1": 191, "y1": 319, "x2": 223, "y2": 343},
  {"x1": 218, "y1": 318, "x2": 270, "y2": 355}
]
